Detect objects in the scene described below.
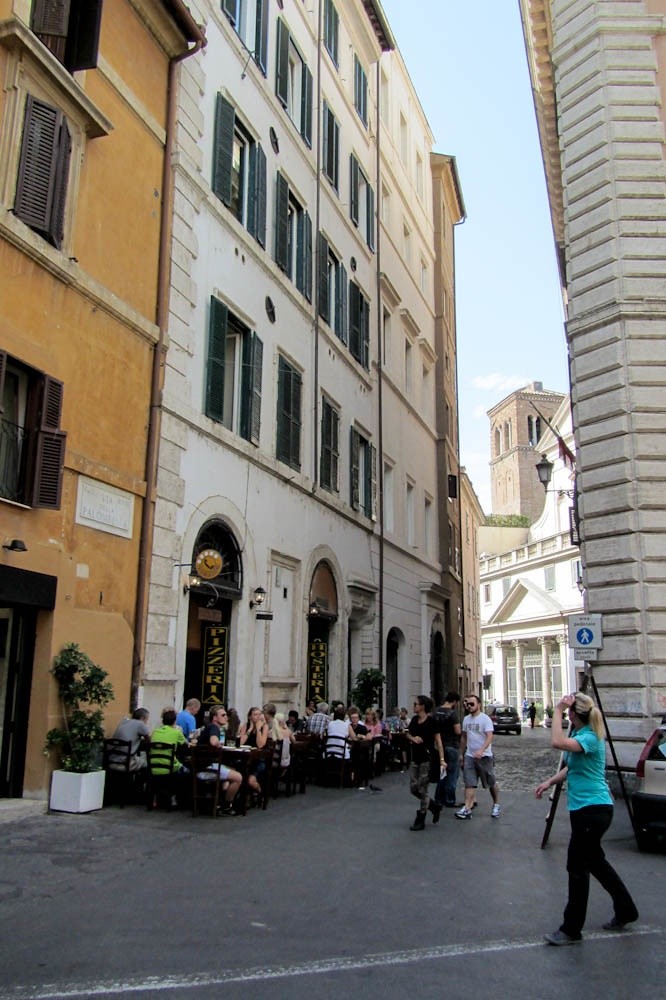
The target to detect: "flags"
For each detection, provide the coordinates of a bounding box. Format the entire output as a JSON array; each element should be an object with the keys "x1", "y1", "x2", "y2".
[{"x1": 557, "y1": 437, "x2": 575, "y2": 471}]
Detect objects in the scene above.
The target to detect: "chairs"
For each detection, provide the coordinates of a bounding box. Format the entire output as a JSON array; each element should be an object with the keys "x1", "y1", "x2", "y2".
[{"x1": 104, "y1": 729, "x2": 405, "y2": 818}]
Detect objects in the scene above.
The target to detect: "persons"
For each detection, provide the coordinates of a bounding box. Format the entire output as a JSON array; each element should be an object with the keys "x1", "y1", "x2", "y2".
[
  {"x1": 156, "y1": 707, "x2": 183, "y2": 732},
  {"x1": 376, "y1": 707, "x2": 411, "y2": 762},
  {"x1": 455, "y1": 695, "x2": 501, "y2": 819},
  {"x1": 108, "y1": 708, "x2": 151, "y2": 789},
  {"x1": 404, "y1": 695, "x2": 447, "y2": 831},
  {"x1": 535, "y1": 694, "x2": 639, "y2": 945},
  {"x1": 522, "y1": 698, "x2": 528, "y2": 720},
  {"x1": 434, "y1": 691, "x2": 468, "y2": 812},
  {"x1": 546, "y1": 706, "x2": 552, "y2": 718},
  {"x1": 287, "y1": 702, "x2": 382, "y2": 781},
  {"x1": 527, "y1": 702, "x2": 536, "y2": 728},
  {"x1": 224, "y1": 703, "x2": 296, "y2": 796},
  {"x1": 197, "y1": 705, "x2": 242, "y2": 817},
  {"x1": 149, "y1": 710, "x2": 191, "y2": 806},
  {"x1": 176, "y1": 699, "x2": 200, "y2": 740}
]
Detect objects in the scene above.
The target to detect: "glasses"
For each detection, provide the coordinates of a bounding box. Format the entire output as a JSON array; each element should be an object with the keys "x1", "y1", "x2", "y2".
[
  {"x1": 414, "y1": 703, "x2": 420, "y2": 707},
  {"x1": 467, "y1": 702, "x2": 480, "y2": 706}
]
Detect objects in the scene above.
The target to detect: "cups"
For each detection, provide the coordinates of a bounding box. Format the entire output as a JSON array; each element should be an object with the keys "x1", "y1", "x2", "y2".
[{"x1": 226, "y1": 740, "x2": 236, "y2": 747}]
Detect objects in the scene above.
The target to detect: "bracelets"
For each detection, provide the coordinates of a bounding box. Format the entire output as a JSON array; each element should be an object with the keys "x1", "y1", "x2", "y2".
[
  {"x1": 245, "y1": 732, "x2": 249, "y2": 736},
  {"x1": 440, "y1": 759, "x2": 444, "y2": 761}
]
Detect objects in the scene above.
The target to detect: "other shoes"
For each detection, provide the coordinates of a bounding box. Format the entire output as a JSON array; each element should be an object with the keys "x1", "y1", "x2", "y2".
[
  {"x1": 603, "y1": 914, "x2": 639, "y2": 929},
  {"x1": 219, "y1": 803, "x2": 236, "y2": 816},
  {"x1": 544, "y1": 930, "x2": 582, "y2": 945},
  {"x1": 492, "y1": 803, "x2": 500, "y2": 818},
  {"x1": 454, "y1": 808, "x2": 472, "y2": 819}
]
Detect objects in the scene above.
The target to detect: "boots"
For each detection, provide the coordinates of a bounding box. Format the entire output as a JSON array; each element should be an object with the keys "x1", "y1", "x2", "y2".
[
  {"x1": 429, "y1": 799, "x2": 443, "y2": 823},
  {"x1": 410, "y1": 810, "x2": 426, "y2": 830}
]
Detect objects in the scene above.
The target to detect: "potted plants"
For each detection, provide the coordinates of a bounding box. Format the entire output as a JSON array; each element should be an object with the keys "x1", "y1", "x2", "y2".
[{"x1": 44, "y1": 643, "x2": 115, "y2": 812}]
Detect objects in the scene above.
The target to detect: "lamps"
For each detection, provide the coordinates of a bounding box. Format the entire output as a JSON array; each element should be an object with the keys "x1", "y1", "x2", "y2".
[
  {"x1": 184, "y1": 569, "x2": 201, "y2": 595},
  {"x1": 306, "y1": 601, "x2": 319, "y2": 622},
  {"x1": 249, "y1": 586, "x2": 267, "y2": 609}
]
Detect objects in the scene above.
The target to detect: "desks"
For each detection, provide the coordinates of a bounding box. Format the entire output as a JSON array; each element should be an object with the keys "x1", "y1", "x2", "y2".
[
  {"x1": 294, "y1": 735, "x2": 382, "y2": 788},
  {"x1": 221, "y1": 745, "x2": 271, "y2": 815}
]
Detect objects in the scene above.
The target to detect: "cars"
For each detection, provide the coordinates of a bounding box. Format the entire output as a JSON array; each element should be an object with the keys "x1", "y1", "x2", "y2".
[
  {"x1": 485, "y1": 704, "x2": 521, "y2": 736},
  {"x1": 631, "y1": 726, "x2": 666, "y2": 854}
]
